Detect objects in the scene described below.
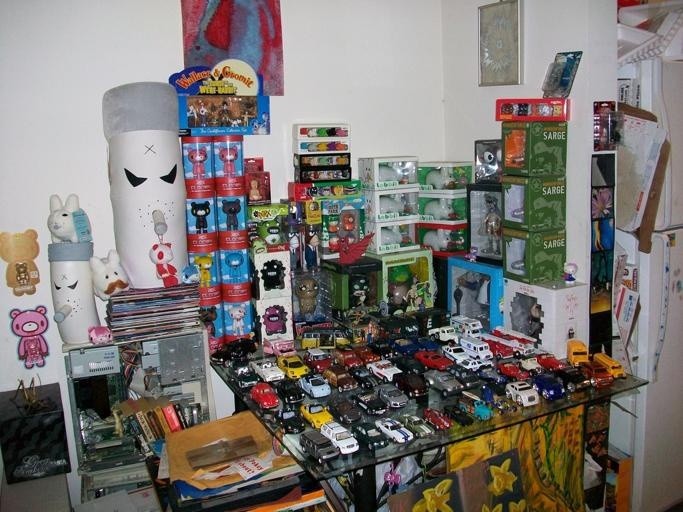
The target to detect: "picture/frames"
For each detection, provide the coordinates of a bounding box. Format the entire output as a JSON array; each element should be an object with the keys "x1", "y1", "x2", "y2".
[{"x1": 477, "y1": 0, "x2": 523, "y2": 87}]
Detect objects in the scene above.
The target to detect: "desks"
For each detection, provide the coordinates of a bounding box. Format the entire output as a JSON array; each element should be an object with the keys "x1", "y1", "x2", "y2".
[{"x1": 208, "y1": 338, "x2": 648, "y2": 512}]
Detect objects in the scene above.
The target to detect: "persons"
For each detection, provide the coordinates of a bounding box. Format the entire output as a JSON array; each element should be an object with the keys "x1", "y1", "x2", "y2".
[{"x1": 483, "y1": 195, "x2": 502, "y2": 256}]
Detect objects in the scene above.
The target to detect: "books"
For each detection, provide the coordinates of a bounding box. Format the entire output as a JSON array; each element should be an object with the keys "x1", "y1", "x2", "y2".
[{"x1": 112, "y1": 398, "x2": 201, "y2": 444}]
